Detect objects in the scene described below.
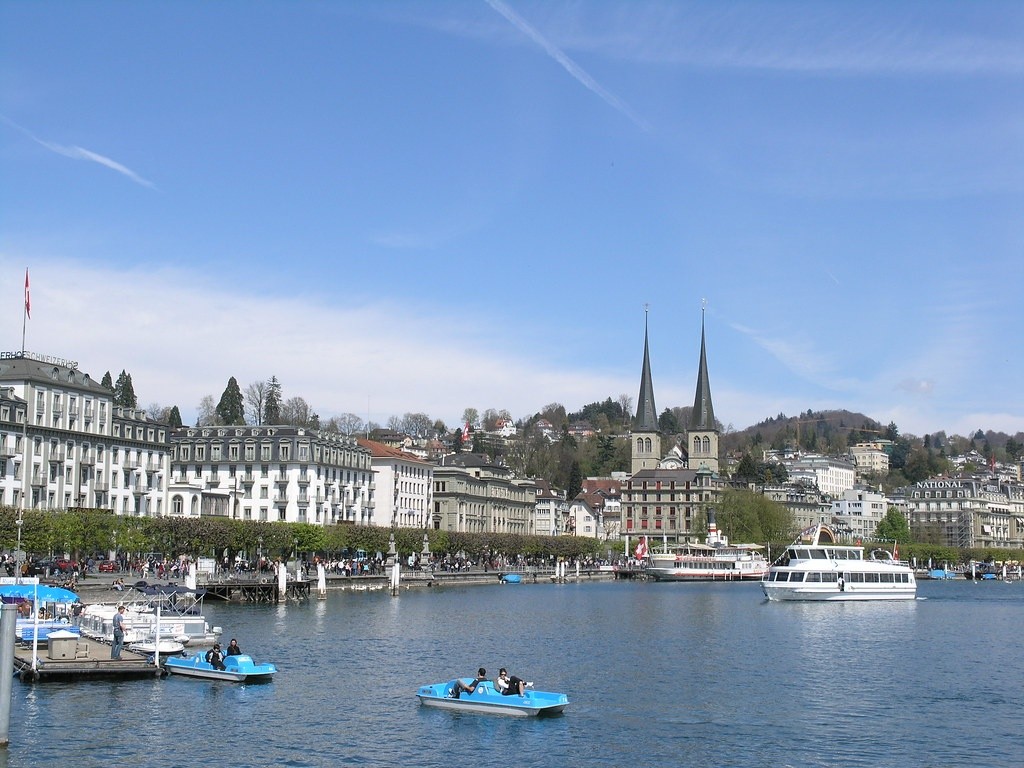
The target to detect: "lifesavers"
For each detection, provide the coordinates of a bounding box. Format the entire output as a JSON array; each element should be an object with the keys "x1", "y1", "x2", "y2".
[
  {"x1": 260, "y1": 578, "x2": 267, "y2": 584},
  {"x1": 676, "y1": 556, "x2": 715, "y2": 562}
]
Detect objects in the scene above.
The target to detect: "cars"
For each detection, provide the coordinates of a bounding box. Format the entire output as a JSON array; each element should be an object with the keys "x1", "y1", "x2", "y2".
[
  {"x1": 30, "y1": 556, "x2": 76, "y2": 577},
  {"x1": 99, "y1": 560, "x2": 121, "y2": 573}
]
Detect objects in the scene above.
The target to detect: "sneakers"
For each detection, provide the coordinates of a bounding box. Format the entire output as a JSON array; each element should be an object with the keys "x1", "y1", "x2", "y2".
[
  {"x1": 523, "y1": 681, "x2": 533, "y2": 687},
  {"x1": 520, "y1": 696, "x2": 524, "y2": 698}
]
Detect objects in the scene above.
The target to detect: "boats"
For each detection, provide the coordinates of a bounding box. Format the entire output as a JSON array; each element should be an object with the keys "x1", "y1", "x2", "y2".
[
  {"x1": 78, "y1": 582, "x2": 223, "y2": 653},
  {"x1": 164, "y1": 650, "x2": 277, "y2": 680},
  {"x1": 415, "y1": 678, "x2": 570, "y2": 716},
  {"x1": 759, "y1": 524, "x2": 917, "y2": 601},
  {"x1": 643, "y1": 543, "x2": 770, "y2": 580}
]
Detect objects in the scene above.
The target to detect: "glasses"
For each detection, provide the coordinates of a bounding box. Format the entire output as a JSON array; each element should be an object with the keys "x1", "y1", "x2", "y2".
[
  {"x1": 500, "y1": 674, "x2": 506, "y2": 675},
  {"x1": 215, "y1": 650, "x2": 220, "y2": 652}
]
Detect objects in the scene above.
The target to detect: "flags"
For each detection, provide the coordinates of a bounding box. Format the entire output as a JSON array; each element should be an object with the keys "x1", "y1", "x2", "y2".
[
  {"x1": 991, "y1": 451, "x2": 994, "y2": 470},
  {"x1": 25, "y1": 269, "x2": 31, "y2": 319},
  {"x1": 894, "y1": 543, "x2": 899, "y2": 561},
  {"x1": 462, "y1": 421, "x2": 470, "y2": 442},
  {"x1": 634, "y1": 536, "x2": 647, "y2": 560},
  {"x1": 855, "y1": 537, "x2": 862, "y2": 547}
]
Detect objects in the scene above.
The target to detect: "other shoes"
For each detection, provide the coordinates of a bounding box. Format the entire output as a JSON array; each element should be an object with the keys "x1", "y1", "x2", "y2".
[
  {"x1": 111, "y1": 656, "x2": 122, "y2": 659},
  {"x1": 448, "y1": 688, "x2": 459, "y2": 697}
]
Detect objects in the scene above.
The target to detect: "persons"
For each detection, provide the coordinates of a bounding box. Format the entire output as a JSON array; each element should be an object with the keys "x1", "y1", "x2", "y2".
[
  {"x1": 111, "y1": 606, "x2": 128, "y2": 659},
  {"x1": 304, "y1": 554, "x2": 386, "y2": 580},
  {"x1": 0, "y1": 548, "x2": 197, "y2": 592},
  {"x1": 38, "y1": 598, "x2": 86, "y2": 623},
  {"x1": 774, "y1": 551, "x2": 790, "y2": 566},
  {"x1": 401, "y1": 552, "x2": 648, "y2": 578},
  {"x1": 912, "y1": 559, "x2": 1024, "y2": 574},
  {"x1": 497, "y1": 668, "x2": 534, "y2": 698},
  {"x1": 227, "y1": 639, "x2": 241, "y2": 656},
  {"x1": 205, "y1": 644, "x2": 226, "y2": 671},
  {"x1": 215, "y1": 554, "x2": 279, "y2": 583},
  {"x1": 838, "y1": 575, "x2": 845, "y2": 591},
  {"x1": 448, "y1": 668, "x2": 487, "y2": 698}
]
